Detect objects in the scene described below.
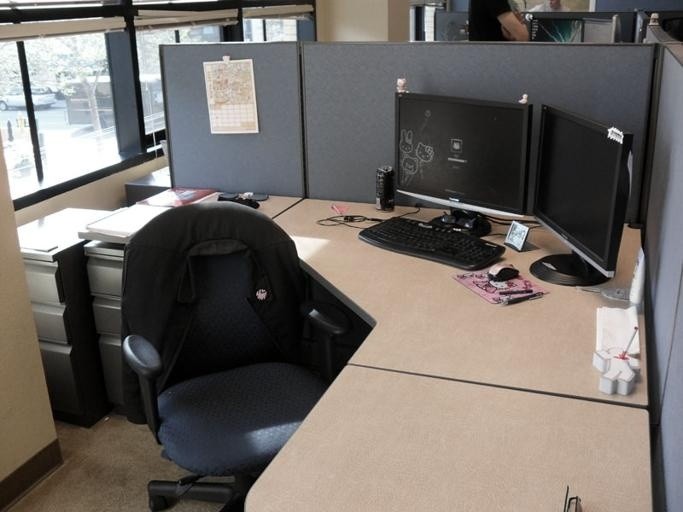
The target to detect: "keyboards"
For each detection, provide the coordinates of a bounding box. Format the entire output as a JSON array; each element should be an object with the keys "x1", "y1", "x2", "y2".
[{"x1": 358, "y1": 217, "x2": 505, "y2": 270}]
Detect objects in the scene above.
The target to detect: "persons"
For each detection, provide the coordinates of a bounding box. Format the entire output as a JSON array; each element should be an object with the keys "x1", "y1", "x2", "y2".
[
  {"x1": 525, "y1": 0, "x2": 571, "y2": 22},
  {"x1": 468, "y1": 0, "x2": 529, "y2": 41}
]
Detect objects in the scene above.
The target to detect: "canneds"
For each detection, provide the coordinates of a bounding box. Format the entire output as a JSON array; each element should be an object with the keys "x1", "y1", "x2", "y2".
[{"x1": 375, "y1": 166, "x2": 394, "y2": 212}]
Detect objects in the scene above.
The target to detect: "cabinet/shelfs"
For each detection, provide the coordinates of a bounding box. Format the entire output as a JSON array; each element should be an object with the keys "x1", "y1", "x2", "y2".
[{"x1": 17, "y1": 208, "x2": 113, "y2": 427}]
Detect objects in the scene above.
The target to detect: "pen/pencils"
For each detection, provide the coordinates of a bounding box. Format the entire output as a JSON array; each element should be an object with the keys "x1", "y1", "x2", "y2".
[
  {"x1": 499, "y1": 289, "x2": 533, "y2": 295},
  {"x1": 619, "y1": 326, "x2": 638, "y2": 358},
  {"x1": 504, "y1": 292, "x2": 544, "y2": 305}
]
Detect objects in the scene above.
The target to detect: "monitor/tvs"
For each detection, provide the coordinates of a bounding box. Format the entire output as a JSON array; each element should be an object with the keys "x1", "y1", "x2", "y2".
[
  {"x1": 529, "y1": 17, "x2": 584, "y2": 43},
  {"x1": 614, "y1": 15, "x2": 621, "y2": 43},
  {"x1": 583, "y1": 18, "x2": 613, "y2": 43},
  {"x1": 394, "y1": 92, "x2": 533, "y2": 238},
  {"x1": 530, "y1": 103, "x2": 634, "y2": 286}
]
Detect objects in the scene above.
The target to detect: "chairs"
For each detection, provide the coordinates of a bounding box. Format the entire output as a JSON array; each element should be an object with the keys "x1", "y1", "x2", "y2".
[{"x1": 121, "y1": 202, "x2": 345, "y2": 509}]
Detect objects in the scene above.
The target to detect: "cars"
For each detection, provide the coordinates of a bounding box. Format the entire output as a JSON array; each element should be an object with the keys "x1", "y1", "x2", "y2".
[{"x1": 1, "y1": 87, "x2": 56, "y2": 111}]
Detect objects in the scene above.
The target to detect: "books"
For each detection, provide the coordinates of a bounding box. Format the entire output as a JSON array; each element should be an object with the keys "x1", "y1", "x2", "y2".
[{"x1": 85, "y1": 202, "x2": 172, "y2": 237}]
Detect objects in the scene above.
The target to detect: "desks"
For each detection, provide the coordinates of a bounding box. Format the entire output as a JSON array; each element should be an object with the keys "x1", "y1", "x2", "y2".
[
  {"x1": 78, "y1": 190, "x2": 301, "y2": 243},
  {"x1": 243, "y1": 364, "x2": 650, "y2": 512},
  {"x1": 270, "y1": 196, "x2": 649, "y2": 407}
]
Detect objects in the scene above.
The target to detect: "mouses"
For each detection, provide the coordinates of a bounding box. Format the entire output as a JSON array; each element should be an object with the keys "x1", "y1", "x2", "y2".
[{"x1": 488, "y1": 263, "x2": 519, "y2": 282}]
